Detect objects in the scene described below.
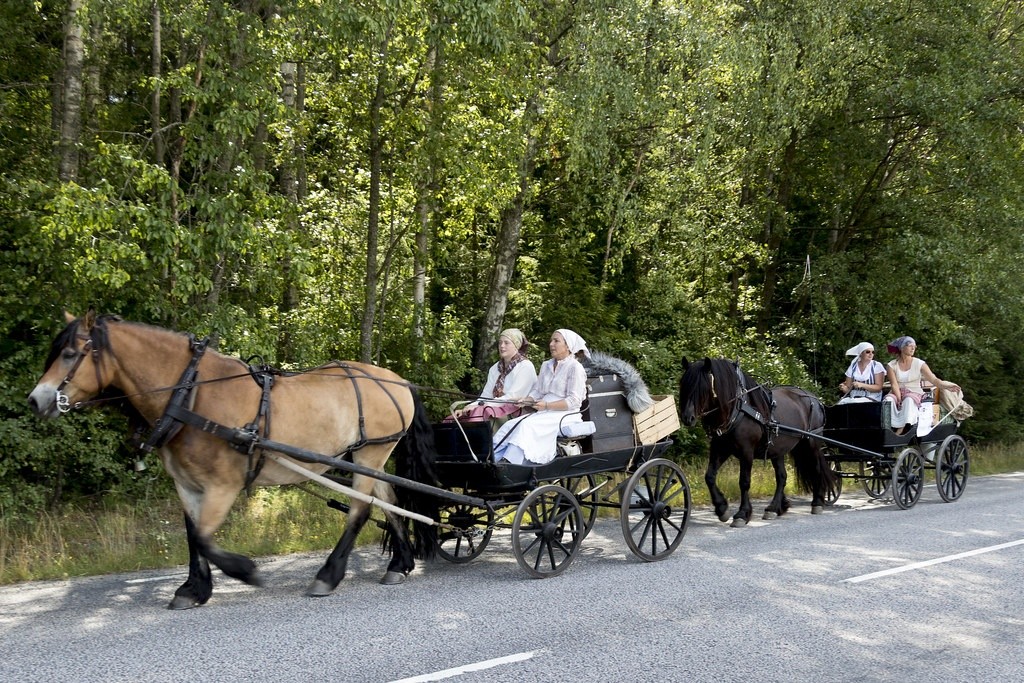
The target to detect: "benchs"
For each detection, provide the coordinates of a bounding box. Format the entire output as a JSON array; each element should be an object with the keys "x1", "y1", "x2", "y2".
[
  {"x1": 918, "y1": 386, "x2": 939, "y2": 421},
  {"x1": 558, "y1": 419, "x2": 598, "y2": 443}
]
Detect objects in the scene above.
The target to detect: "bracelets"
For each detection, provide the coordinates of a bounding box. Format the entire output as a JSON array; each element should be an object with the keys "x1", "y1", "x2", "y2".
[{"x1": 545, "y1": 402, "x2": 548, "y2": 412}]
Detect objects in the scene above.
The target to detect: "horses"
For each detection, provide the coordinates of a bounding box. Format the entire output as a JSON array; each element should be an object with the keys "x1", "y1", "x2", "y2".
[
  {"x1": 679, "y1": 356, "x2": 840, "y2": 527},
  {"x1": 28, "y1": 311, "x2": 443, "y2": 611}
]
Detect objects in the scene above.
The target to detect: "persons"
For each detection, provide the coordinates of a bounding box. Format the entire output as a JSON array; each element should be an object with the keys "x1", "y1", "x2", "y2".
[
  {"x1": 836, "y1": 342, "x2": 888, "y2": 405},
  {"x1": 443, "y1": 328, "x2": 538, "y2": 436},
  {"x1": 882, "y1": 336, "x2": 960, "y2": 435},
  {"x1": 492, "y1": 329, "x2": 590, "y2": 464}
]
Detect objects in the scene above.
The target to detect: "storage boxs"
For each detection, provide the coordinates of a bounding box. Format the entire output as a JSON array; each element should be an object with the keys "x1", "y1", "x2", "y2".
[{"x1": 631, "y1": 393, "x2": 682, "y2": 445}]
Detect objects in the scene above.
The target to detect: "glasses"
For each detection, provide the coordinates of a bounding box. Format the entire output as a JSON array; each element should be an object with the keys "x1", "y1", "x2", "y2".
[
  {"x1": 906, "y1": 345, "x2": 917, "y2": 349},
  {"x1": 864, "y1": 350, "x2": 875, "y2": 354}
]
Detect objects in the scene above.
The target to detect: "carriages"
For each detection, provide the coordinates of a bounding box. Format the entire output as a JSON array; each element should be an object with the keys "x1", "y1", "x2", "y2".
[
  {"x1": 676, "y1": 353, "x2": 970, "y2": 528},
  {"x1": 22, "y1": 306, "x2": 696, "y2": 611}
]
